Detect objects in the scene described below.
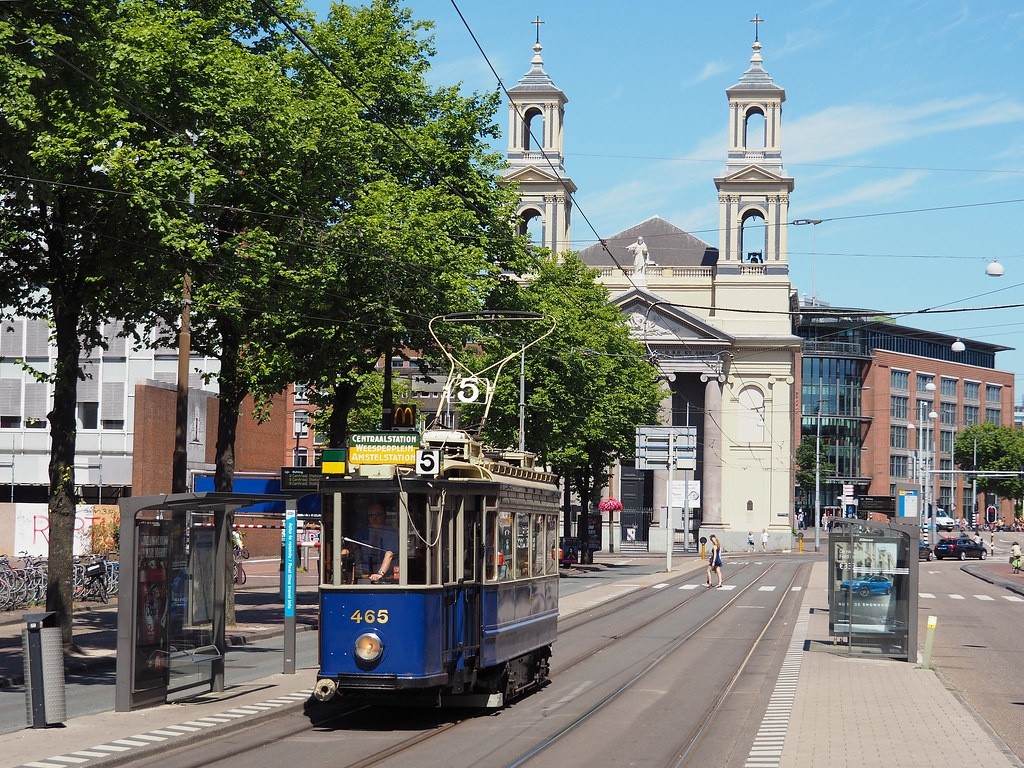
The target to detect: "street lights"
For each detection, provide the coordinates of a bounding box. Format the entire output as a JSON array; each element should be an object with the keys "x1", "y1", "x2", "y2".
[{"x1": 924, "y1": 409, "x2": 938, "y2": 525}]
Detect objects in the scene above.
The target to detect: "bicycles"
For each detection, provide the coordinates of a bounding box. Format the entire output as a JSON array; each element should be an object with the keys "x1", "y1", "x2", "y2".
[
  {"x1": 1010, "y1": 552, "x2": 1020, "y2": 574},
  {"x1": 232, "y1": 545, "x2": 250, "y2": 585},
  {"x1": 0, "y1": 550, "x2": 119, "y2": 612}
]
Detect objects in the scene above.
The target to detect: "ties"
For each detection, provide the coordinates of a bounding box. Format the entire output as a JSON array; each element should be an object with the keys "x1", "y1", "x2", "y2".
[{"x1": 371, "y1": 530, "x2": 379, "y2": 564}]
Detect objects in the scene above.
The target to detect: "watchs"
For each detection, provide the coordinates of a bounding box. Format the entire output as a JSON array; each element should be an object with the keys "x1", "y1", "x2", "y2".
[{"x1": 378, "y1": 570, "x2": 385, "y2": 575}]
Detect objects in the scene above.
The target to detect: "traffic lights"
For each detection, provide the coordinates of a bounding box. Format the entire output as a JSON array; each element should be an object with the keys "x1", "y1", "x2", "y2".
[{"x1": 988, "y1": 506, "x2": 997, "y2": 523}]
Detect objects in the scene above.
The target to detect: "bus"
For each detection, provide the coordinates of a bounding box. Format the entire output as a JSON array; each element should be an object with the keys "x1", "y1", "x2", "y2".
[
  {"x1": 303, "y1": 429, "x2": 560, "y2": 725},
  {"x1": 856, "y1": 494, "x2": 932, "y2": 522}
]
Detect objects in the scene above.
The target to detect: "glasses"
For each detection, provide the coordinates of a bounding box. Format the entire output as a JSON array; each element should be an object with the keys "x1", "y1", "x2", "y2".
[{"x1": 367, "y1": 512, "x2": 386, "y2": 517}]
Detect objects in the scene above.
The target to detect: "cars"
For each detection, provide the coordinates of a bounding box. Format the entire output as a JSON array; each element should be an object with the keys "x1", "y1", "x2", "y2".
[
  {"x1": 919, "y1": 539, "x2": 933, "y2": 561},
  {"x1": 840, "y1": 574, "x2": 892, "y2": 597},
  {"x1": 934, "y1": 537, "x2": 988, "y2": 561}
]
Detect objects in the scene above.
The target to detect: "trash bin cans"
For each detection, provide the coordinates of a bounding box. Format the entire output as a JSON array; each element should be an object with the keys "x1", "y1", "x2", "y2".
[{"x1": 21, "y1": 612, "x2": 67, "y2": 727}]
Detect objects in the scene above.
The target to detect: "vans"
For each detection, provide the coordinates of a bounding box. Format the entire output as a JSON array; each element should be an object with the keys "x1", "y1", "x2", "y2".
[{"x1": 928, "y1": 508, "x2": 956, "y2": 532}]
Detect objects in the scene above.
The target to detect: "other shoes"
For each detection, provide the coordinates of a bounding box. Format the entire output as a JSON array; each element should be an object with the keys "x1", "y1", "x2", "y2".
[
  {"x1": 703, "y1": 584, "x2": 710, "y2": 588},
  {"x1": 717, "y1": 584, "x2": 722, "y2": 587},
  {"x1": 714, "y1": 585, "x2": 719, "y2": 588}
]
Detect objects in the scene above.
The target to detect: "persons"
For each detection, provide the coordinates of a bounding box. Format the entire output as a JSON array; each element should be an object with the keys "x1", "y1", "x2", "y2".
[
  {"x1": 760, "y1": 528, "x2": 769, "y2": 553},
  {"x1": 230, "y1": 526, "x2": 248, "y2": 557},
  {"x1": 958, "y1": 529, "x2": 984, "y2": 547},
  {"x1": 955, "y1": 516, "x2": 966, "y2": 531},
  {"x1": 979, "y1": 515, "x2": 1024, "y2": 532},
  {"x1": 747, "y1": 531, "x2": 755, "y2": 553},
  {"x1": 1009, "y1": 542, "x2": 1022, "y2": 570},
  {"x1": 341, "y1": 504, "x2": 399, "y2": 585},
  {"x1": 623, "y1": 236, "x2": 648, "y2": 277},
  {"x1": 702, "y1": 535, "x2": 722, "y2": 588},
  {"x1": 798, "y1": 512, "x2": 866, "y2": 533}
]
